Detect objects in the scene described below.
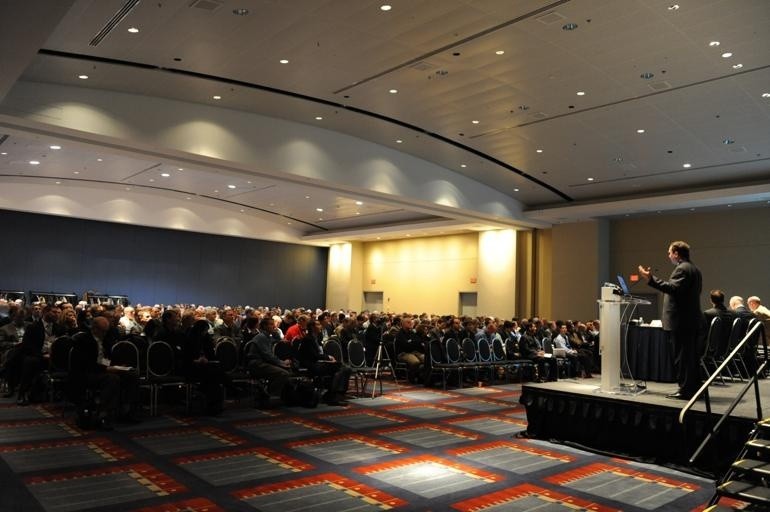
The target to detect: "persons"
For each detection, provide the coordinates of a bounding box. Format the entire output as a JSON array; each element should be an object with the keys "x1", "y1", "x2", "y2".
[
  {"x1": 637, "y1": 239, "x2": 704, "y2": 401},
  {"x1": 746, "y1": 295, "x2": 770, "y2": 346},
  {"x1": 728, "y1": 295, "x2": 760, "y2": 345},
  {"x1": 704, "y1": 289, "x2": 737, "y2": 359}
]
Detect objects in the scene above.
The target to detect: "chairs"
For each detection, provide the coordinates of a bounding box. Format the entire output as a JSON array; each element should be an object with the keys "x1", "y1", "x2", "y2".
[
  {"x1": 696, "y1": 316, "x2": 767, "y2": 385},
  {"x1": 1, "y1": 290, "x2": 600, "y2": 417}
]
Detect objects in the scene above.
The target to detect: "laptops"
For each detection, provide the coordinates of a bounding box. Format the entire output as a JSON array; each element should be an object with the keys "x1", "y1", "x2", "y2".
[{"x1": 616, "y1": 273, "x2": 646, "y2": 299}]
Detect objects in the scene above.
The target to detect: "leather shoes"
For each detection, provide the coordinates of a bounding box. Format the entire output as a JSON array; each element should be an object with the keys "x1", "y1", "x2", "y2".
[
  {"x1": 16, "y1": 391, "x2": 25, "y2": 404},
  {"x1": 95, "y1": 415, "x2": 114, "y2": 433},
  {"x1": 117, "y1": 414, "x2": 144, "y2": 424},
  {"x1": 666, "y1": 390, "x2": 703, "y2": 401}
]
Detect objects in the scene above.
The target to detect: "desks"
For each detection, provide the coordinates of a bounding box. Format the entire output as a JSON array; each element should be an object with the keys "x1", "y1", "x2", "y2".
[{"x1": 620, "y1": 320, "x2": 715, "y2": 384}]
